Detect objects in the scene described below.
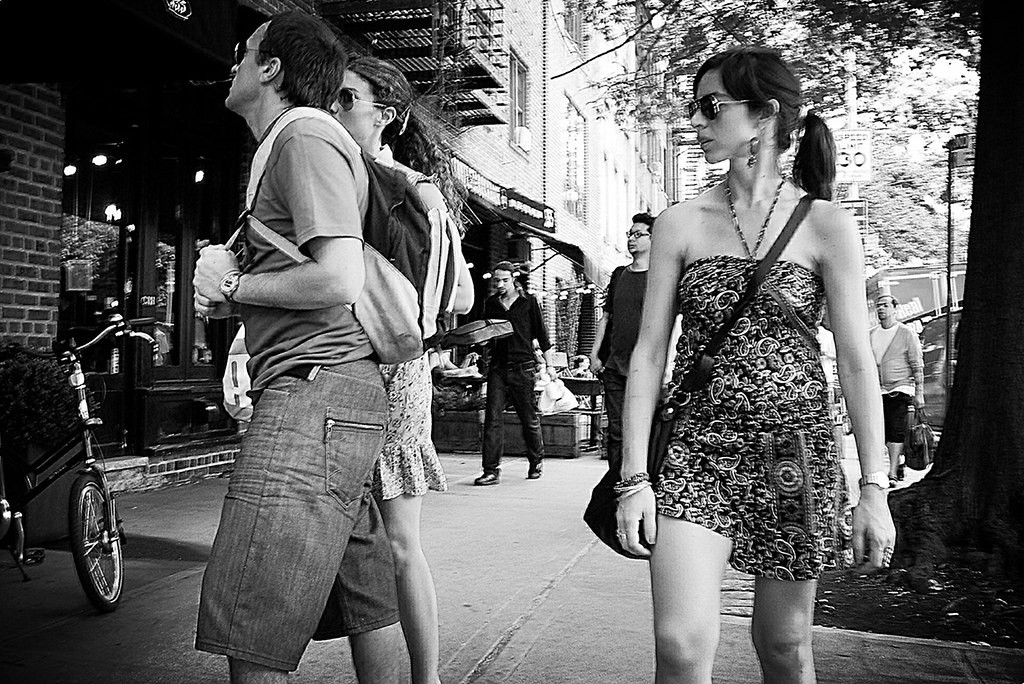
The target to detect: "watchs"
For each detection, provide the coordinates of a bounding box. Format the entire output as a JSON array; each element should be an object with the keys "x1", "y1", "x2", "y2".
[
  {"x1": 858, "y1": 471, "x2": 890, "y2": 492},
  {"x1": 219, "y1": 269, "x2": 244, "y2": 306}
]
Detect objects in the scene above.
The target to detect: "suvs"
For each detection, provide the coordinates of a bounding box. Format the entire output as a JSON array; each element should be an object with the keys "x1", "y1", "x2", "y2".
[{"x1": 918, "y1": 308, "x2": 964, "y2": 433}]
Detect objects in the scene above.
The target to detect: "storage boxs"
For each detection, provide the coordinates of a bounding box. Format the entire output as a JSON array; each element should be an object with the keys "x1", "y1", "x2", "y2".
[{"x1": 433, "y1": 408, "x2": 580, "y2": 458}]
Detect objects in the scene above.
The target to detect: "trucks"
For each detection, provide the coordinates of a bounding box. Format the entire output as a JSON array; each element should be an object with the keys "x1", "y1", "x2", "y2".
[{"x1": 865, "y1": 262, "x2": 968, "y2": 333}]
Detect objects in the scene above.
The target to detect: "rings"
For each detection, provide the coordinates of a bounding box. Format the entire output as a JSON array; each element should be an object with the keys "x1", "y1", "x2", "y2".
[
  {"x1": 883, "y1": 546, "x2": 894, "y2": 568},
  {"x1": 615, "y1": 528, "x2": 627, "y2": 541}
]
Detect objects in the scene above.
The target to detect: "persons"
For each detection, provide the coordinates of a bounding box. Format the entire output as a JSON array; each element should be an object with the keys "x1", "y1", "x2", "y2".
[
  {"x1": 474, "y1": 262, "x2": 557, "y2": 487},
  {"x1": 191, "y1": 12, "x2": 401, "y2": 684},
  {"x1": 590, "y1": 213, "x2": 655, "y2": 467},
  {"x1": 324, "y1": 58, "x2": 474, "y2": 684},
  {"x1": 609, "y1": 44, "x2": 898, "y2": 684},
  {"x1": 871, "y1": 295, "x2": 923, "y2": 488}
]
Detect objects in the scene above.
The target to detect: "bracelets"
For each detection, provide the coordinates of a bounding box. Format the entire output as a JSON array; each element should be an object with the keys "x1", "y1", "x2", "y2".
[
  {"x1": 546, "y1": 365, "x2": 552, "y2": 367},
  {"x1": 613, "y1": 472, "x2": 651, "y2": 501}
]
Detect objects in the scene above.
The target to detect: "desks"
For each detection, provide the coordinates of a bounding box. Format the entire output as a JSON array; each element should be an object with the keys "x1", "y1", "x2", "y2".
[{"x1": 559, "y1": 377, "x2": 605, "y2": 452}]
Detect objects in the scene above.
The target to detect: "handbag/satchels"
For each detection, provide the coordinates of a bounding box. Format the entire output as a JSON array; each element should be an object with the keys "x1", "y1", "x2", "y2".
[
  {"x1": 533, "y1": 379, "x2": 579, "y2": 417},
  {"x1": 904, "y1": 408, "x2": 936, "y2": 470},
  {"x1": 582, "y1": 404, "x2": 677, "y2": 559}
]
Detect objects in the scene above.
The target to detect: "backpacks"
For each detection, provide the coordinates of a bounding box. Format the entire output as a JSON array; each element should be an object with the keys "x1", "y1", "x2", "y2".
[{"x1": 195, "y1": 106, "x2": 459, "y2": 365}]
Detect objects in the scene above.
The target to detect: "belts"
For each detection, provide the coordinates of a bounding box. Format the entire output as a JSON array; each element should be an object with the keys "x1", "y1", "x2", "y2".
[{"x1": 279, "y1": 363, "x2": 322, "y2": 381}]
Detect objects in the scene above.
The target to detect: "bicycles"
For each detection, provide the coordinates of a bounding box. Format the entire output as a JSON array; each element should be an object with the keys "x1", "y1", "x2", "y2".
[{"x1": 0, "y1": 313, "x2": 157, "y2": 612}]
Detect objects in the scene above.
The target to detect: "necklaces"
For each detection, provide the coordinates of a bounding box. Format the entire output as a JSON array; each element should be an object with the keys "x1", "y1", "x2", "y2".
[{"x1": 725, "y1": 171, "x2": 786, "y2": 275}]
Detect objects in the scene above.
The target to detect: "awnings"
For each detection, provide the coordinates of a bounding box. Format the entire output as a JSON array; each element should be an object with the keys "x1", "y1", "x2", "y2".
[{"x1": 465, "y1": 190, "x2": 590, "y2": 273}]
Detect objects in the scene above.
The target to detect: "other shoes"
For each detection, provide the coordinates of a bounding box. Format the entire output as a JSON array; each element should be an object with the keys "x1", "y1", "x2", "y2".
[
  {"x1": 897, "y1": 470, "x2": 904, "y2": 480},
  {"x1": 473, "y1": 470, "x2": 500, "y2": 486},
  {"x1": 528, "y1": 469, "x2": 542, "y2": 479},
  {"x1": 888, "y1": 475, "x2": 896, "y2": 487}
]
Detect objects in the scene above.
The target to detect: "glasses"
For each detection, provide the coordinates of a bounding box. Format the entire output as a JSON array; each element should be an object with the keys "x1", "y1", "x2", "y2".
[
  {"x1": 688, "y1": 92, "x2": 759, "y2": 119},
  {"x1": 626, "y1": 230, "x2": 650, "y2": 238},
  {"x1": 335, "y1": 86, "x2": 403, "y2": 123},
  {"x1": 234, "y1": 42, "x2": 275, "y2": 66}
]
